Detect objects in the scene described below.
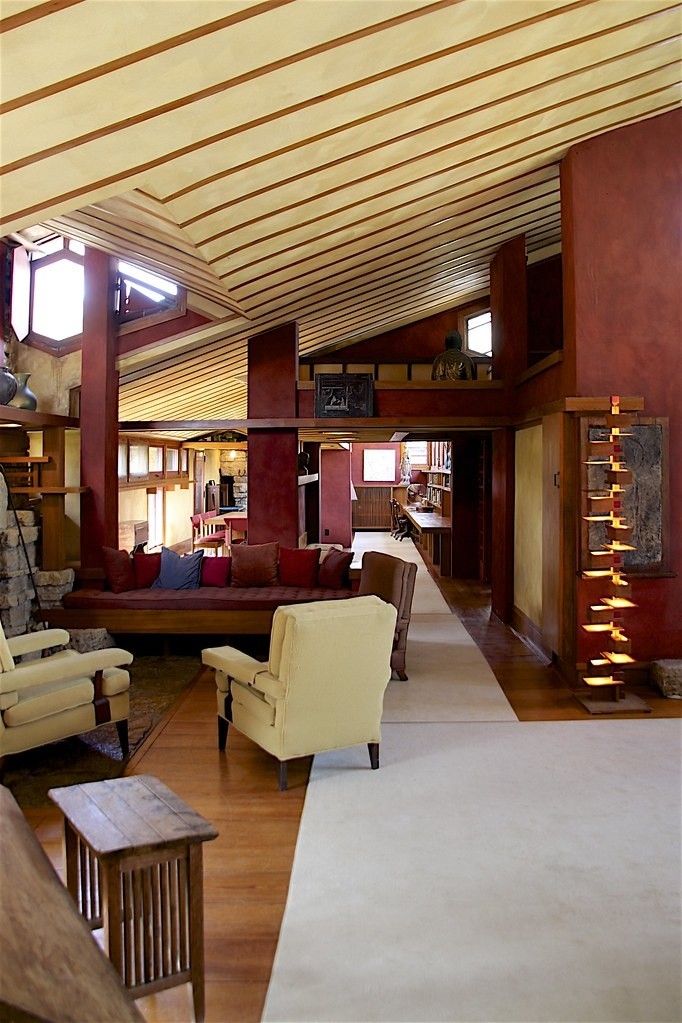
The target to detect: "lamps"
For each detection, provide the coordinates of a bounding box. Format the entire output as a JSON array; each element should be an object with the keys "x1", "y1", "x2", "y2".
[{"x1": 573, "y1": 397, "x2": 654, "y2": 716}]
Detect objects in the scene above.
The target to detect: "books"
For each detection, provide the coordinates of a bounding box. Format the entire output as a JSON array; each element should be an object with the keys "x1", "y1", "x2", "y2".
[
  {"x1": 427, "y1": 473, "x2": 446, "y2": 487},
  {"x1": 426, "y1": 487, "x2": 441, "y2": 507}
]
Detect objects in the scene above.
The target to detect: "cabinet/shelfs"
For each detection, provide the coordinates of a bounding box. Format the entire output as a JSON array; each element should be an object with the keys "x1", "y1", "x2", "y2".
[{"x1": 407, "y1": 441, "x2": 452, "y2": 575}]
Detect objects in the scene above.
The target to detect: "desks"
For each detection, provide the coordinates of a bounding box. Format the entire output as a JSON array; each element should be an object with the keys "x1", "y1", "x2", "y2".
[{"x1": 205, "y1": 512, "x2": 247, "y2": 557}]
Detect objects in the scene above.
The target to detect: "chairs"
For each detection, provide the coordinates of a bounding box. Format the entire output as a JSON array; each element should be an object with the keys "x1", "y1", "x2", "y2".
[
  {"x1": 190, "y1": 510, "x2": 248, "y2": 557},
  {"x1": 202, "y1": 595, "x2": 398, "y2": 790},
  {"x1": 389, "y1": 497, "x2": 413, "y2": 541},
  {"x1": 0, "y1": 621, "x2": 133, "y2": 756}
]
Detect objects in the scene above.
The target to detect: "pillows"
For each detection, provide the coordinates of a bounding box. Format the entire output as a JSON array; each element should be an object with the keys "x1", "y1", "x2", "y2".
[
  {"x1": 230, "y1": 541, "x2": 280, "y2": 586},
  {"x1": 151, "y1": 546, "x2": 205, "y2": 590},
  {"x1": 319, "y1": 547, "x2": 355, "y2": 587},
  {"x1": 184, "y1": 553, "x2": 232, "y2": 588},
  {"x1": 102, "y1": 545, "x2": 135, "y2": 592},
  {"x1": 278, "y1": 546, "x2": 321, "y2": 587},
  {"x1": 134, "y1": 552, "x2": 163, "y2": 588}
]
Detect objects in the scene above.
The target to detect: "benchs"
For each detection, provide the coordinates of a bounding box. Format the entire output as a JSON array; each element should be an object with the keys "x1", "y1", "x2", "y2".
[{"x1": 31, "y1": 560, "x2": 416, "y2": 681}]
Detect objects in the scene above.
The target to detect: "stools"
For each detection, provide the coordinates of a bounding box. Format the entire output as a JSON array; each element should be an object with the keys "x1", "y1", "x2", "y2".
[
  {"x1": 49, "y1": 774, "x2": 219, "y2": 1023},
  {"x1": 305, "y1": 542, "x2": 343, "y2": 564}
]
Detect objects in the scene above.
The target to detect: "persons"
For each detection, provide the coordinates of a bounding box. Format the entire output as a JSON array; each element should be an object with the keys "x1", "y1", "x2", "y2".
[
  {"x1": 298, "y1": 452, "x2": 310, "y2": 476},
  {"x1": 398, "y1": 451, "x2": 412, "y2": 486},
  {"x1": 431, "y1": 331, "x2": 478, "y2": 380}
]
